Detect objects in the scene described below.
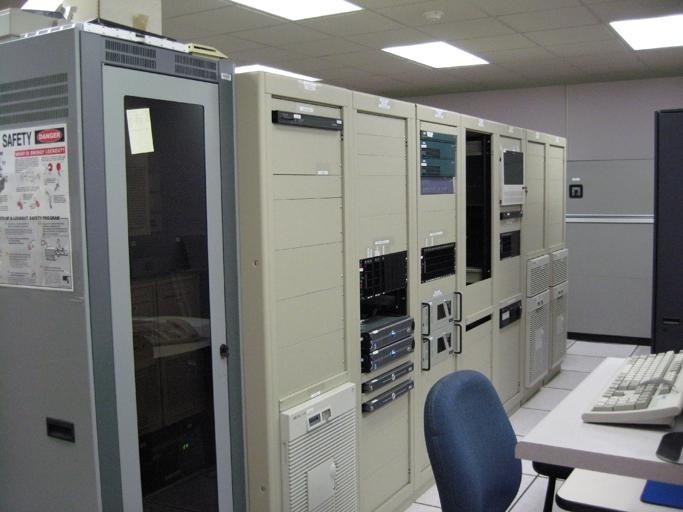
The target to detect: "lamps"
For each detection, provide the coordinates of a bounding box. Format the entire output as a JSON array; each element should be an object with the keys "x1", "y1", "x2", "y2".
[{"x1": 423, "y1": 370, "x2": 574, "y2": 511}]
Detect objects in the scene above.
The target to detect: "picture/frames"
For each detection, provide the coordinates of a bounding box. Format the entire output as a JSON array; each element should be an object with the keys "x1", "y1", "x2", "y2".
[{"x1": 514, "y1": 355, "x2": 682, "y2": 511}]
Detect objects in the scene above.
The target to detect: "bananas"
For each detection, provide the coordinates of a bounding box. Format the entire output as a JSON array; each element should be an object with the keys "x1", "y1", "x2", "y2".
[{"x1": 582, "y1": 349, "x2": 683, "y2": 428}]
